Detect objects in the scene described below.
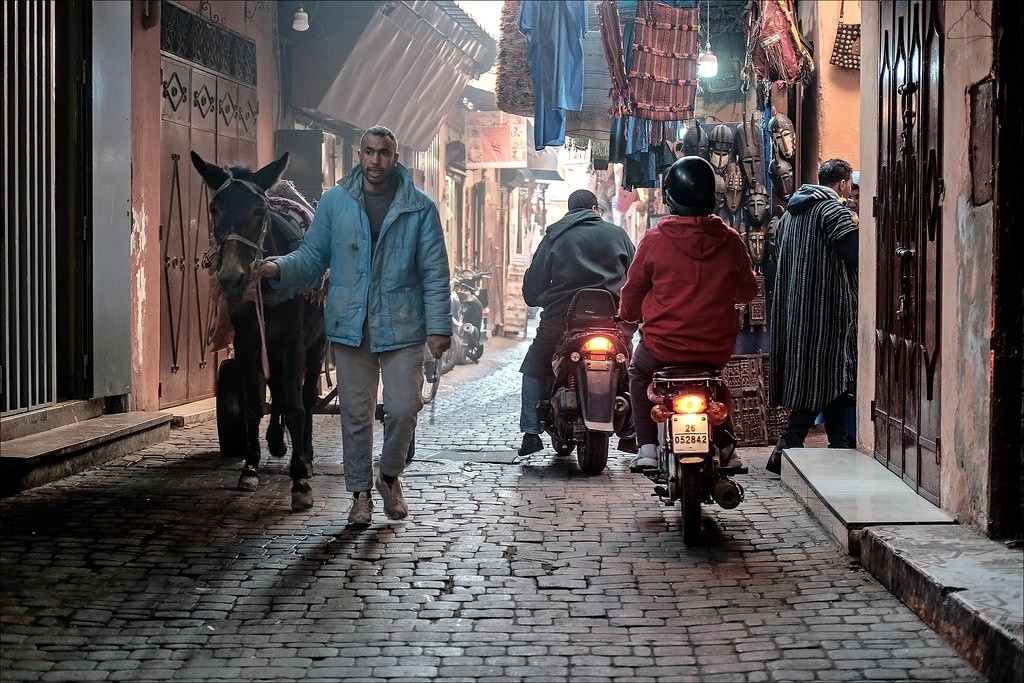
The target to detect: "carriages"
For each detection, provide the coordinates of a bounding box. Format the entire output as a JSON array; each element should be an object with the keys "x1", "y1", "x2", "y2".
[{"x1": 190, "y1": 149, "x2": 442, "y2": 512}]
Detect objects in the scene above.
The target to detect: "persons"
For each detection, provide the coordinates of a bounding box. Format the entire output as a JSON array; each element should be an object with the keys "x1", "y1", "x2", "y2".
[
  {"x1": 252, "y1": 126, "x2": 451, "y2": 522},
  {"x1": 618, "y1": 155, "x2": 760, "y2": 472},
  {"x1": 766, "y1": 158, "x2": 859, "y2": 475},
  {"x1": 517, "y1": 187, "x2": 635, "y2": 454}
]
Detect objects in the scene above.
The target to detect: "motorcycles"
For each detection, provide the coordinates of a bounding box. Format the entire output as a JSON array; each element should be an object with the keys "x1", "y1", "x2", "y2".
[
  {"x1": 535, "y1": 287, "x2": 632, "y2": 474},
  {"x1": 440, "y1": 268, "x2": 493, "y2": 364},
  {"x1": 608, "y1": 310, "x2": 749, "y2": 546}
]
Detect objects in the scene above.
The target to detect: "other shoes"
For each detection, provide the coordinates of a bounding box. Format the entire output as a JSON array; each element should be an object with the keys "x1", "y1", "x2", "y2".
[
  {"x1": 618, "y1": 437, "x2": 639, "y2": 453},
  {"x1": 765, "y1": 435, "x2": 788, "y2": 476},
  {"x1": 517, "y1": 434, "x2": 544, "y2": 457},
  {"x1": 629, "y1": 443, "x2": 659, "y2": 470},
  {"x1": 719, "y1": 444, "x2": 744, "y2": 470}
]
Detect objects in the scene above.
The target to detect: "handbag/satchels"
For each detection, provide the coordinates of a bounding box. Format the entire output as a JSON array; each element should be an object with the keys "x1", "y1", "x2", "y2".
[{"x1": 829, "y1": 23, "x2": 860, "y2": 70}]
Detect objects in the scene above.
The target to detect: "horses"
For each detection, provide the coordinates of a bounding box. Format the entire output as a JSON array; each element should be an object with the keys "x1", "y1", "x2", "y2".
[{"x1": 189, "y1": 148, "x2": 330, "y2": 512}]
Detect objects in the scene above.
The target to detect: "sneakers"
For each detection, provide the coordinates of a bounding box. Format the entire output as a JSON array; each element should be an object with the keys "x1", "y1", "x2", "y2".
[
  {"x1": 375, "y1": 474, "x2": 410, "y2": 521},
  {"x1": 347, "y1": 492, "x2": 375, "y2": 527}
]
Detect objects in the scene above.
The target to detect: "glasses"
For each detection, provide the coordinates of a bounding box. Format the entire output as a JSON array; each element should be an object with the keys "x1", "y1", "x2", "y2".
[{"x1": 596, "y1": 206, "x2": 605, "y2": 218}]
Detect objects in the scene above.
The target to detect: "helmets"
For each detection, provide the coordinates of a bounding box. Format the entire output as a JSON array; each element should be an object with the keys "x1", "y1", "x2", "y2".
[{"x1": 661, "y1": 155, "x2": 716, "y2": 218}]
